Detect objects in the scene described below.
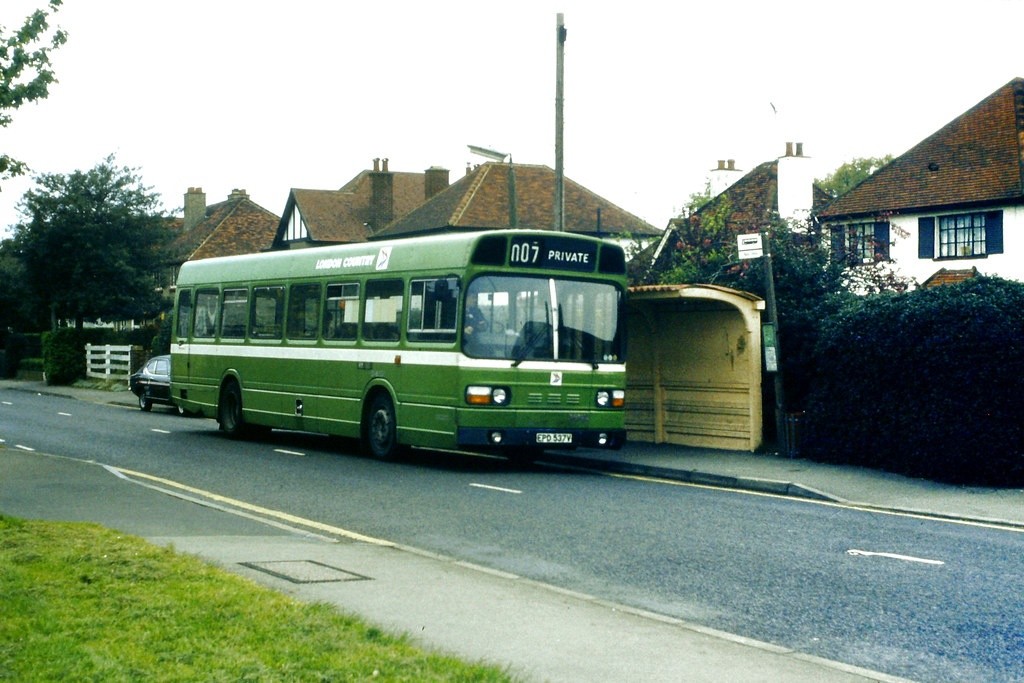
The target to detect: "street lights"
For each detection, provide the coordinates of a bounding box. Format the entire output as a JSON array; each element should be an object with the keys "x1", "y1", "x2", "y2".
[{"x1": 467, "y1": 143, "x2": 517, "y2": 228}]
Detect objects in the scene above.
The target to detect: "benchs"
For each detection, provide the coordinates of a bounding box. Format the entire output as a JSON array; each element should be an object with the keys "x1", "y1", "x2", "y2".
[
  {"x1": 339, "y1": 322, "x2": 400, "y2": 342},
  {"x1": 220, "y1": 322, "x2": 244, "y2": 336}
]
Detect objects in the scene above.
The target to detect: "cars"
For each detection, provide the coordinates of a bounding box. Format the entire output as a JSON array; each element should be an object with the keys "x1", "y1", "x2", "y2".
[{"x1": 130, "y1": 355, "x2": 189, "y2": 417}]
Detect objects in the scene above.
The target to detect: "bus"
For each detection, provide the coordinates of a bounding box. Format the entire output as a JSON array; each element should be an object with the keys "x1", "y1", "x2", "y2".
[{"x1": 169, "y1": 226, "x2": 630, "y2": 465}]
